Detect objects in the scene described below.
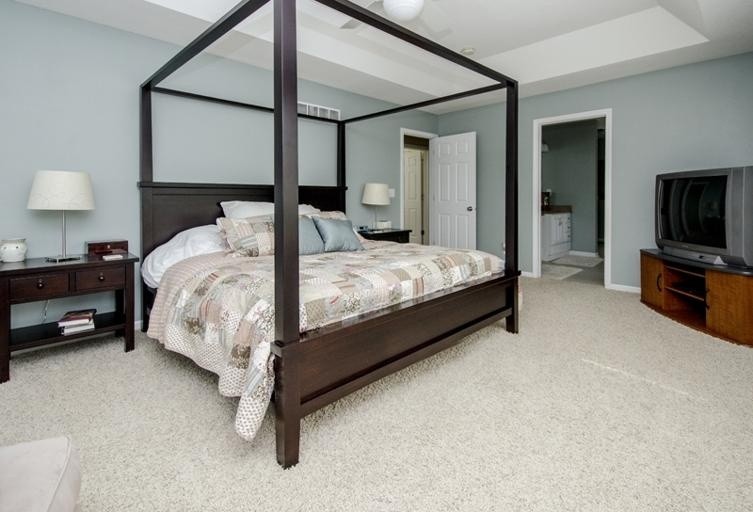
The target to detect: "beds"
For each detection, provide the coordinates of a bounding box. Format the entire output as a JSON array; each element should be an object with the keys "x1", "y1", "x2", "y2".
[{"x1": 137, "y1": 0, "x2": 523, "y2": 468}]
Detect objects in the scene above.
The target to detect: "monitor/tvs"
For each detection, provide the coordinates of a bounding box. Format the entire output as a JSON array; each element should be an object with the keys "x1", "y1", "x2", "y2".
[{"x1": 655, "y1": 166, "x2": 753, "y2": 269}]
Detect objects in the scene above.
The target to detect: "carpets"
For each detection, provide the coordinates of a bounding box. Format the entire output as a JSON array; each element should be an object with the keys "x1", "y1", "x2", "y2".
[{"x1": 540, "y1": 263, "x2": 583, "y2": 281}]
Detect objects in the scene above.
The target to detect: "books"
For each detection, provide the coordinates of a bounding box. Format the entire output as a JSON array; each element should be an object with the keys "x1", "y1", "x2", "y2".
[
  {"x1": 60, "y1": 317, "x2": 97, "y2": 337},
  {"x1": 56, "y1": 308, "x2": 97, "y2": 327}
]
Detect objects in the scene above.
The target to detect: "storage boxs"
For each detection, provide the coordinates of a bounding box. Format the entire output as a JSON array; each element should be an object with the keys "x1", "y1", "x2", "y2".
[{"x1": 84, "y1": 239, "x2": 128, "y2": 257}]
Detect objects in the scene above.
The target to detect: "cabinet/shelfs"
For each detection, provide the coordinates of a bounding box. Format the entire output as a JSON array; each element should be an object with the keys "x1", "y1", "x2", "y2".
[
  {"x1": 638, "y1": 246, "x2": 751, "y2": 352},
  {"x1": 541, "y1": 213, "x2": 571, "y2": 261}
]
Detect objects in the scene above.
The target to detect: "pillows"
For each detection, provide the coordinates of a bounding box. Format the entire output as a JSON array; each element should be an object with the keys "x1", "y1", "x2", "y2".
[
  {"x1": 219, "y1": 212, "x2": 275, "y2": 257},
  {"x1": 298, "y1": 215, "x2": 325, "y2": 255},
  {"x1": 321, "y1": 209, "x2": 368, "y2": 243},
  {"x1": 312, "y1": 213, "x2": 364, "y2": 252},
  {"x1": 219, "y1": 199, "x2": 319, "y2": 217}
]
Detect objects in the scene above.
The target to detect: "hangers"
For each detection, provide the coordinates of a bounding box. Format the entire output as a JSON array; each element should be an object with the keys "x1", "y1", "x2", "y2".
[{"x1": 552, "y1": 255, "x2": 602, "y2": 268}]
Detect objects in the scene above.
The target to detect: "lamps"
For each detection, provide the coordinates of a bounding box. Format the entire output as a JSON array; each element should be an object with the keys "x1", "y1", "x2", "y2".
[
  {"x1": 361, "y1": 181, "x2": 390, "y2": 230},
  {"x1": 383, "y1": 0, "x2": 425, "y2": 21},
  {"x1": 26, "y1": 170, "x2": 94, "y2": 262}
]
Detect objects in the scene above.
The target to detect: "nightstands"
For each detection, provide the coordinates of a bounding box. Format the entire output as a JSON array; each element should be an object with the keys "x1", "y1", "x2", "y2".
[
  {"x1": 0, "y1": 248, "x2": 140, "y2": 385},
  {"x1": 357, "y1": 226, "x2": 411, "y2": 243}
]
